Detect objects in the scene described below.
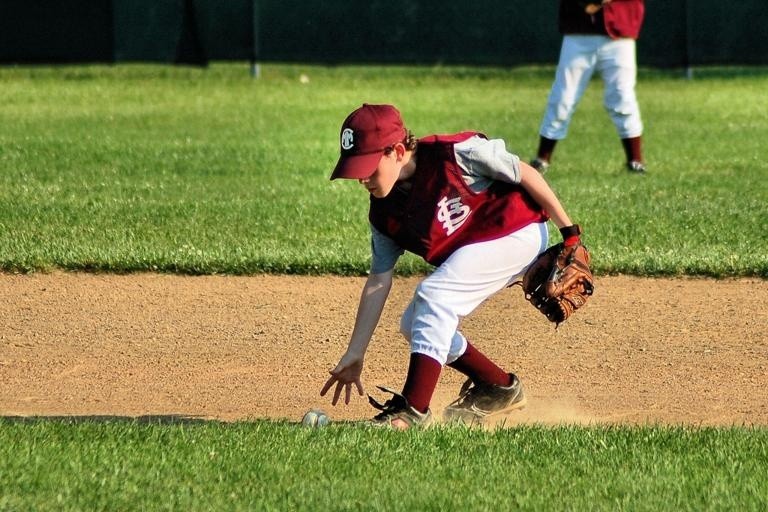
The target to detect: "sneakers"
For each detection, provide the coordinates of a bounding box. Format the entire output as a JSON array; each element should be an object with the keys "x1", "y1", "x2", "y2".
[
  {"x1": 366, "y1": 387, "x2": 434, "y2": 432},
  {"x1": 528, "y1": 157, "x2": 551, "y2": 176},
  {"x1": 624, "y1": 160, "x2": 648, "y2": 173},
  {"x1": 441, "y1": 371, "x2": 528, "y2": 423}
]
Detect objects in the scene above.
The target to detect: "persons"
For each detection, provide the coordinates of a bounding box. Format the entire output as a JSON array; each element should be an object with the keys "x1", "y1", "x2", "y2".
[
  {"x1": 531, "y1": 0, "x2": 649, "y2": 173},
  {"x1": 315, "y1": 103, "x2": 582, "y2": 433}
]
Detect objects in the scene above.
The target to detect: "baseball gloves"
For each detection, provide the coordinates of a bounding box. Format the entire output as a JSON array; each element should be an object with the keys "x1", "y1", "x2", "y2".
[{"x1": 507, "y1": 244, "x2": 593, "y2": 329}]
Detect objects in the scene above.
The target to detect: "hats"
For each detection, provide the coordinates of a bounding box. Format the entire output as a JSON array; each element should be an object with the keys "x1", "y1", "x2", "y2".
[{"x1": 330, "y1": 104, "x2": 407, "y2": 180}]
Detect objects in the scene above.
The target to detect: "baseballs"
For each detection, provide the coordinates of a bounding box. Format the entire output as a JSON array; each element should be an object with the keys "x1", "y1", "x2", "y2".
[{"x1": 302, "y1": 410, "x2": 327, "y2": 426}]
